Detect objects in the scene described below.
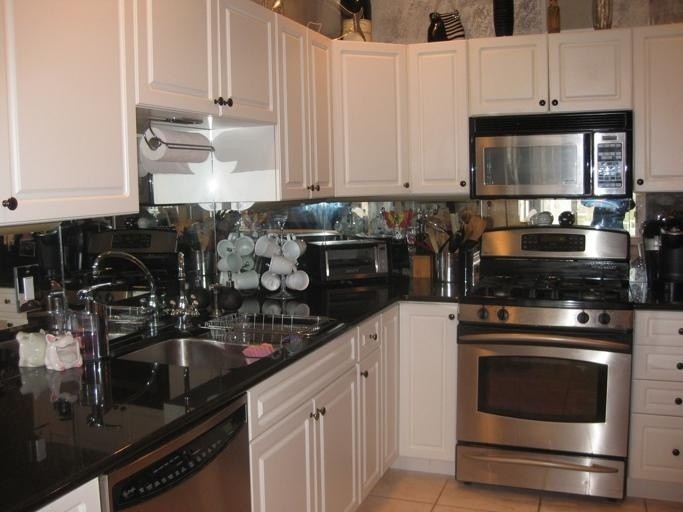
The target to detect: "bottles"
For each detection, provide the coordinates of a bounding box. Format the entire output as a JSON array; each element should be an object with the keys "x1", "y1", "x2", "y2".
[
  {"x1": 70, "y1": 282, "x2": 112, "y2": 358},
  {"x1": 338, "y1": 1, "x2": 374, "y2": 43},
  {"x1": 593, "y1": 1, "x2": 614, "y2": 30},
  {"x1": 492, "y1": 1, "x2": 515, "y2": 37},
  {"x1": 78, "y1": 360, "x2": 116, "y2": 429},
  {"x1": 640, "y1": 215, "x2": 683, "y2": 307},
  {"x1": 548, "y1": 1, "x2": 560, "y2": 34}
]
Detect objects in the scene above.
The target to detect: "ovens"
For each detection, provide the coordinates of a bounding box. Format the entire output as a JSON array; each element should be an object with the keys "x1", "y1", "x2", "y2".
[{"x1": 455, "y1": 330, "x2": 634, "y2": 500}]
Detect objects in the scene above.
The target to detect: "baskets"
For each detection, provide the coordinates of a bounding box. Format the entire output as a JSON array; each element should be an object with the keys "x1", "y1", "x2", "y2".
[{"x1": 202, "y1": 312, "x2": 327, "y2": 346}]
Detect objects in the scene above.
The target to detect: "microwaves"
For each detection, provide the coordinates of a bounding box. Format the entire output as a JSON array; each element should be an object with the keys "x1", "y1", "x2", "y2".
[
  {"x1": 306, "y1": 241, "x2": 389, "y2": 283},
  {"x1": 467, "y1": 111, "x2": 631, "y2": 199}
]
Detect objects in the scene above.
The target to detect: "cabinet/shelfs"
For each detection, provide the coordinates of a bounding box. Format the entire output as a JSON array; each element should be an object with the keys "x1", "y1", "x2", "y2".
[
  {"x1": 352, "y1": 303, "x2": 398, "y2": 506},
  {"x1": 207, "y1": 15, "x2": 334, "y2": 202},
  {"x1": 630, "y1": 309, "x2": 682, "y2": 506},
  {"x1": 130, "y1": 0, "x2": 278, "y2": 128},
  {"x1": 33, "y1": 473, "x2": 102, "y2": 512},
  {"x1": 0, "y1": 1, "x2": 140, "y2": 229},
  {"x1": 405, "y1": 39, "x2": 470, "y2": 204},
  {"x1": 394, "y1": 300, "x2": 459, "y2": 476},
  {"x1": 326, "y1": 40, "x2": 412, "y2": 201},
  {"x1": 468, "y1": 27, "x2": 634, "y2": 118},
  {"x1": 631, "y1": 20, "x2": 681, "y2": 192},
  {"x1": 247, "y1": 328, "x2": 363, "y2": 512}
]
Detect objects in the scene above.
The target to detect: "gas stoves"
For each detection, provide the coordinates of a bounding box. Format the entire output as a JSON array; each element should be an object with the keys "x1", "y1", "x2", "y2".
[{"x1": 458, "y1": 271, "x2": 634, "y2": 332}]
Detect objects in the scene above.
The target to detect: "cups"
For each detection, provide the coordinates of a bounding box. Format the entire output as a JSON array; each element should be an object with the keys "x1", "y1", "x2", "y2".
[
  {"x1": 214, "y1": 236, "x2": 311, "y2": 290},
  {"x1": 235, "y1": 299, "x2": 311, "y2": 317},
  {"x1": 434, "y1": 252, "x2": 463, "y2": 281}
]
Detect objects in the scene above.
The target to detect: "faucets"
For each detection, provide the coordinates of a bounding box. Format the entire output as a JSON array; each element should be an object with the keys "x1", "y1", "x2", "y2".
[
  {"x1": 182, "y1": 367, "x2": 192, "y2": 414},
  {"x1": 177, "y1": 252, "x2": 189, "y2": 309},
  {"x1": 91, "y1": 251, "x2": 163, "y2": 327},
  {"x1": 120, "y1": 363, "x2": 158, "y2": 405}
]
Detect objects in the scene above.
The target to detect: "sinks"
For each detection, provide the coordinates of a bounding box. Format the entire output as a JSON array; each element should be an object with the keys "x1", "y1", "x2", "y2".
[
  {"x1": 106, "y1": 325, "x2": 141, "y2": 347},
  {"x1": 117, "y1": 338, "x2": 283, "y2": 370},
  {"x1": 93, "y1": 289, "x2": 151, "y2": 301}
]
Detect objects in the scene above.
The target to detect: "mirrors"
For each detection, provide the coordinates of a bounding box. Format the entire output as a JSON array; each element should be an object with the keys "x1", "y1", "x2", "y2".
[{"x1": 482, "y1": 190, "x2": 637, "y2": 235}]
